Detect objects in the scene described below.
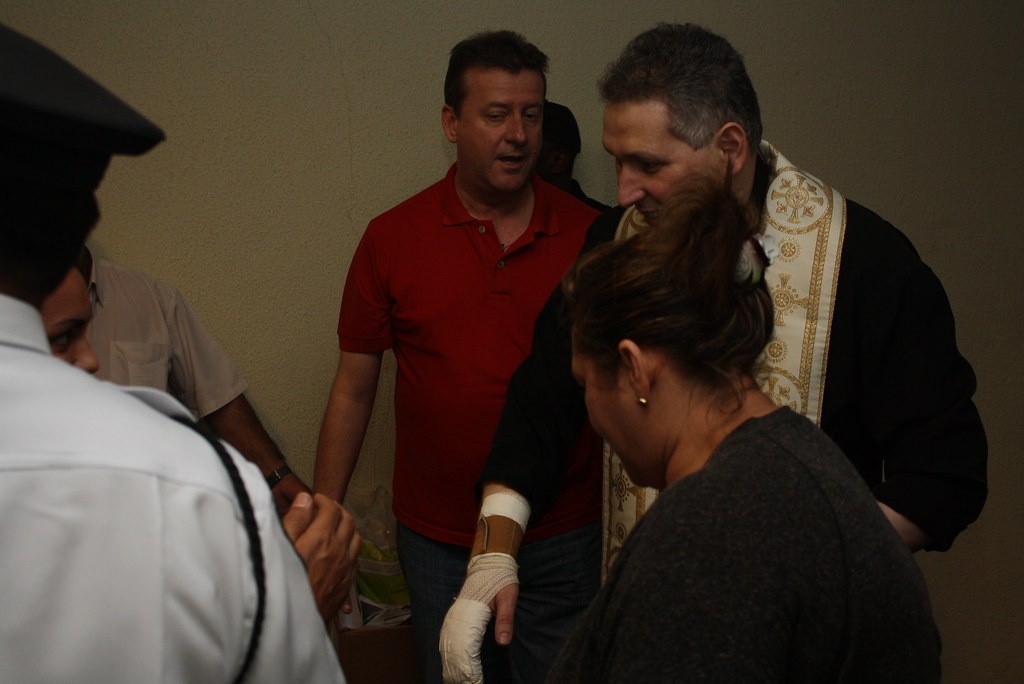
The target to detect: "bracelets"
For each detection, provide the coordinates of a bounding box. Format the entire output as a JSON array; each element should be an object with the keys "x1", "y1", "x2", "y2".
[{"x1": 266, "y1": 465, "x2": 292, "y2": 489}]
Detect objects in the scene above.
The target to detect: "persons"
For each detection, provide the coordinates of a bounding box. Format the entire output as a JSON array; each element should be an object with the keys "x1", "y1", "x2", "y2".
[
  {"x1": 1, "y1": 22, "x2": 363, "y2": 684},
  {"x1": 440, "y1": 21, "x2": 987, "y2": 684},
  {"x1": 18, "y1": 255, "x2": 100, "y2": 374},
  {"x1": 82, "y1": 248, "x2": 312, "y2": 516},
  {"x1": 534, "y1": 101, "x2": 614, "y2": 214},
  {"x1": 545, "y1": 174, "x2": 944, "y2": 684},
  {"x1": 312, "y1": 29, "x2": 601, "y2": 684}
]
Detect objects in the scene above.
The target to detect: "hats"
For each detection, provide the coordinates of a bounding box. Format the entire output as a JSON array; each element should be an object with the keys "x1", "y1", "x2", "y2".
[
  {"x1": 0, "y1": 25, "x2": 167, "y2": 154},
  {"x1": 543, "y1": 100, "x2": 581, "y2": 155}
]
